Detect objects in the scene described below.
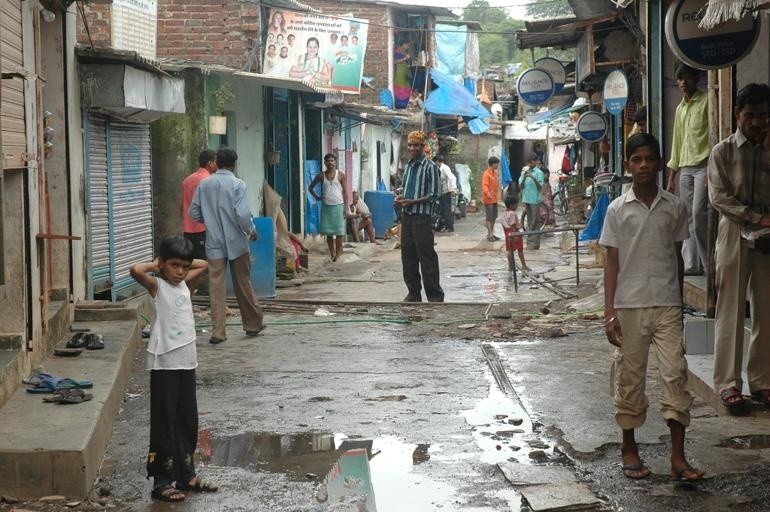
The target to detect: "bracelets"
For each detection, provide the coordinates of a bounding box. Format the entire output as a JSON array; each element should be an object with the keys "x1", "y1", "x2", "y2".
[{"x1": 605, "y1": 316, "x2": 617, "y2": 325}]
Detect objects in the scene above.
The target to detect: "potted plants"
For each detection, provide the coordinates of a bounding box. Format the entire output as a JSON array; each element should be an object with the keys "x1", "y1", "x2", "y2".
[{"x1": 209, "y1": 80, "x2": 237, "y2": 135}]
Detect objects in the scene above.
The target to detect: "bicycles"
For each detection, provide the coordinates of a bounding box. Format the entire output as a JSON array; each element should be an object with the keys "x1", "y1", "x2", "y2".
[{"x1": 520, "y1": 169, "x2": 620, "y2": 240}]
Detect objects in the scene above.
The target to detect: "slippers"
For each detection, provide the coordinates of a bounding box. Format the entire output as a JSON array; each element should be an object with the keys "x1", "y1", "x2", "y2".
[
  {"x1": 209, "y1": 337, "x2": 228, "y2": 344},
  {"x1": 84, "y1": 333, "x2": 104, "y2": 349},
  {"x1": 41, "y1": 387, "x2": 74, "y2": 402},
  {"x1": 66, "y1": 333, "x2": 87, "y2": 348},
  {"x1": 246, "y1": 323, "x2": 266, "y2": 336},
  {"x1": 26, "y1": 380, "x2": 63, "y2": 393},
  {"x1": 142, "y1": 324, "x2": 151, "y2": 337},
  {"x1": 23, "y1": 373, "x2": 61, "y2": 385},
  {"x1": 55, "y1": 378, "x2": 92, "y2": 389},
  {"x1": 57, "y1": 389, "x2": 92, "y2": 404},
  {"x1": 487, "y1": 236, "x2": 497, "y2": 242},
  {"x1": 494, "y1": 236, "x2": 500, "y2": 240},
  {"x1": 672, "y1": 464, "x2": 704, "y2": 481},
  {"x1": 623, "y1": 461, "x2": 650, "y2": 478}
]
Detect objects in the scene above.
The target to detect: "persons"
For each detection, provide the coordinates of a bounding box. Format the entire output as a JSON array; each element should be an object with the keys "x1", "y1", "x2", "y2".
[
  {"x1": 666, "y1": 65, "x2": 708, "y2": 276},
  {"x1": 393, "y1": 131, "x2": 555, "y2": 303},
  {"x1": 188, "y1": 147, "x2": 267, "y2": 344},
  {"x1": 599, "y1": 133, "x2": 705, "y2": 484},
  {"x1": 309, "y1": 153, "x2": 351, "y2": 262},
  {"x1": 347, "y1": 191, "x2": 383, "y2": 245},
  {"x1": 181, "y1": 150, "x2": 236, "y2": 316},
  {"x1": 631, "y1": 105, "x2": 646, "y2": 132},
  {"x1": 266, "y1": 10, "x2": 358, "y2": 86},
  {"x1": 130, "y1": 235, "x2": 218, "y2": 501},
  {"x1": 707, "y1": 83, "x2": 770, "y2": 407},
  {"x1": 394, "y1": 37, "x2": 413, "y2": 108}
]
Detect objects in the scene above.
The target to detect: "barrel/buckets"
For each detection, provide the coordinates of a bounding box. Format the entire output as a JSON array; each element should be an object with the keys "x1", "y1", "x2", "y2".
[
  {"x1": 362, "y1": 190, "x2": 395, "y2": 240},
  {"x1": 225, "y1": 217, "x2": 278, "y2": 298}
]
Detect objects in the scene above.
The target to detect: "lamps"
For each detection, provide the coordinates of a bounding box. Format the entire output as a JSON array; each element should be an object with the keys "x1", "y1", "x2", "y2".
[{"x1": 38, "y1": 0, "x2": 56, "y2": 23}]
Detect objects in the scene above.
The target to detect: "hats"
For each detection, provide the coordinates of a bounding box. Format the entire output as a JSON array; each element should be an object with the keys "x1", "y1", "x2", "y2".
[{"x1": 408, "y1": 130, "x2": 427, "y2": 143}]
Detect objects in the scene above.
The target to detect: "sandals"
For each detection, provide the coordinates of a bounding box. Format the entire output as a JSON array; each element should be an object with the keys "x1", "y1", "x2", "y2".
[
  {"x1": 721, "y1": 388, "x2": 744, "y2": 406},
  {"x1": 150, "y1": 484, "x2": 185, "y2": 502},
  {"x1": 751, "y1": 390, "x2": 770, "y2": 406},
  {"x1": 176, "y1": 476, "x2": 218, "y2": 494}
]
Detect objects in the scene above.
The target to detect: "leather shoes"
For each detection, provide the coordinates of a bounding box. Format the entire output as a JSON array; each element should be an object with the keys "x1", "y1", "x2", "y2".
[{"x1": 444, "y1": 228, "x2": 454, "y2": 233}]
